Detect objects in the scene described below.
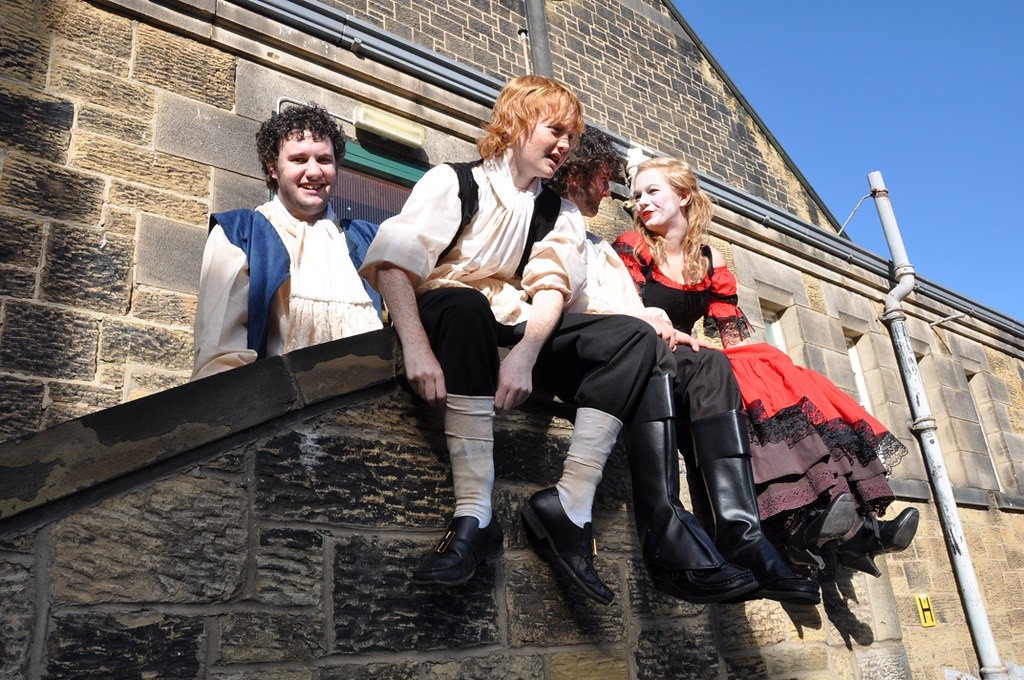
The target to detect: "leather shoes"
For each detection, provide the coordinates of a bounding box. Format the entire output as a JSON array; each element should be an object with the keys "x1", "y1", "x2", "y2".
[
  {"x1": 408, "y1": 507, "x2": 504, "y2": 587},
  {"x1": 520, "y1": 486, "x2": 616, "y2": 606}
]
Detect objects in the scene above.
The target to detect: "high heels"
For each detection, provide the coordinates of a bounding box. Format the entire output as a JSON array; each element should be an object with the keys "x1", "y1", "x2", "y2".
[
  {"x1": 836, "y1": 507, "x2": 919, "y2": 578},
  {"x1": 787, "y1": 491, "x2": 856, "y2": 570}
]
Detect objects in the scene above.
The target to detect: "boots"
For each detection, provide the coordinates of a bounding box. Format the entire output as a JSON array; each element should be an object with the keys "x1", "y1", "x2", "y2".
[
  {"x1": 681, "y1": 408, "x2": 821, "y2": 605},
  {"x1": 628, "y1": 373, "x2": 760, "y2": 604}
]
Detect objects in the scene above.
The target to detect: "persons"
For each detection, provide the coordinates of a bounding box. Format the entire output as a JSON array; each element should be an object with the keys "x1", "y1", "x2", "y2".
[
  {"x1": 542, "y1": 123, "x2": 821, "y2": 608},
  {"x1": 190, "y1": 103, "x2": 395, "y2": 383},
  {"x1": 612, "y1": 155, "x2": 920, "y2": 596},
  {"x1": 358, "y1": 76, "x2": 658, "y2": 606}
]
